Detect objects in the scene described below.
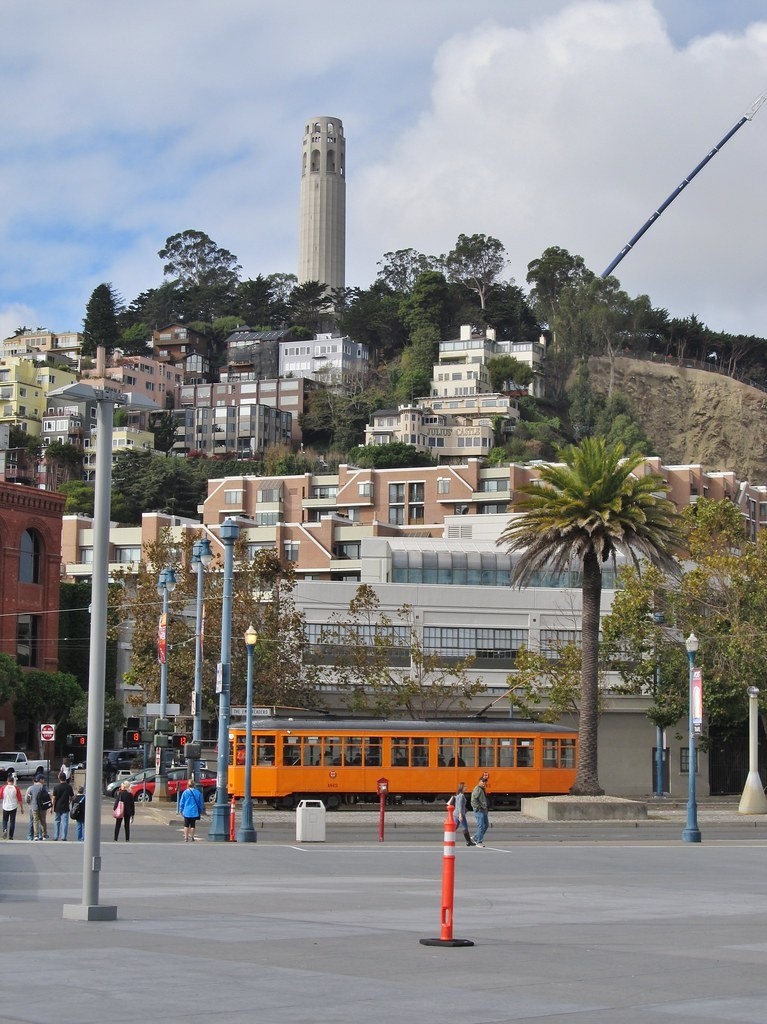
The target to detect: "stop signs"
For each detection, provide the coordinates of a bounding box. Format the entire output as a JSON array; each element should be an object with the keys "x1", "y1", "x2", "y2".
[{"x1": 42, "y1": 725, "x2": 54, "y2": 740}]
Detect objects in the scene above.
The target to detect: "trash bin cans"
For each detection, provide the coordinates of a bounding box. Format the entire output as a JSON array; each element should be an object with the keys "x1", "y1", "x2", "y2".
[
  {"x1": 116, "y1": 770, "x2": 132, "y2": 781},
  {"x1": 296, "y1": 799, "x2": 326, "y2": 843}
]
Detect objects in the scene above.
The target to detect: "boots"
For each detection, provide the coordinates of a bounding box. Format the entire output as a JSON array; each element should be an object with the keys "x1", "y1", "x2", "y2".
[{"x1": 465, "y1": 833, "x2": 475, "y2": 846}]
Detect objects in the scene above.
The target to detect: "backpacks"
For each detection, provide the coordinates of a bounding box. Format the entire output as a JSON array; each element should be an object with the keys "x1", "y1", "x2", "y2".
[{"x1": 37, "y1": 788, "x2": 53, "y2": 809}]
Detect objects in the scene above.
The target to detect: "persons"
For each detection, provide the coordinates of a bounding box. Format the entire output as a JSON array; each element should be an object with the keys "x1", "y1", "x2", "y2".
[
  {"x1": 49, "y1": 773, "x2": 73, "y2": 841},
  {"x1": 113, "y1": 781, "x2": 135, "y2": 843},
  {"x1": 71, "y1": 786, "x2": 85, "y2": 842},
  {"x1": 26, "y1": 779, "x2": 42, "y2": 840},
  {"x1": 452, "y1": 782, "x2": 475, "y2": 847},
  {"x1": 29, "y1": 774, "x2": 51, "y2": 841},
  {"x1": 0, "y1": 777, "x2": 23, "y2": 840},
  {"x1": 179, "y1": 779, "x2": 204, "y2": 842},
  {"x1": 102, "y1": 753, "x2": 117, "y2": 795},
  {"x1": 60, "y1": 758, "x2": 71, "y2": 785},
  {"x1": 263, "y1": 747, "x2": 466, "y2": 768},
  {"x1": 470, "y1": 777, "x2": 488, "y2": 847}
]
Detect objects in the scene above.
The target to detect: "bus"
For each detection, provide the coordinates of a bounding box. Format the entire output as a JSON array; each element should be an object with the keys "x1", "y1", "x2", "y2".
[{"x1": 227, "y1": 718, "x2": 579, "y2": 810}]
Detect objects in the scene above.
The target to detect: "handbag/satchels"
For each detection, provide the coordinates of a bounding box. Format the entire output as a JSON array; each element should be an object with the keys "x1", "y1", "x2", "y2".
[
  {"x1": 70, "y1": 803, "x2": 80, "y2": 819},
  {"x1": 198, "y1": 807, "x2": 202, "y2": 814},
  {"x1": 113, "y1": 792, "x2": 123, "y2": 818},
  {"x1": 447, "y1": 796, "x2": 456, "y2": 807}
]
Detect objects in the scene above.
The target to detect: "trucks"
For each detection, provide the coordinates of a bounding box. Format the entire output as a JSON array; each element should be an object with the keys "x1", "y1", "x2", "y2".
[{"x1": 0, "y1": 752, "x2": 51, "y2": 781}]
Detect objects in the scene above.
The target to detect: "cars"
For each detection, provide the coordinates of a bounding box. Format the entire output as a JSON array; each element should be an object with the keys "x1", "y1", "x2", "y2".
[
  {"x1": 130, "y1": 767, "x2": 217, "y2": 803},
  {"x1": 107, "y1": 749, "x2": 143, "y2": 774},
  {"x1": 105, "y1": 767, "x2": 170, "y2": 800}
]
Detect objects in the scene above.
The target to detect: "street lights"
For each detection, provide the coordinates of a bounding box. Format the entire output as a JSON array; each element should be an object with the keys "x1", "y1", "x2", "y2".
[
  {"x1": 653, "y1": 611, "x2": 665, "y2": 799},
  {"x1": 189, "y1": 536, "x2": 217, "y2": 786},
  {"x1": 237, "y1": 621, "x2": 257, "y2": 842},
  {"x1": 682, "y1": 633, "x2": 702, "y2": 842},
  {"x1": 151, "y1": 566, "x2": 177, "y2": 802},
  {"x1": 204, "y1": 519, "x2": 240, "y2": 841},
  {"x1": 47, "y1": 381, "x2": 160, "y2": 919}
]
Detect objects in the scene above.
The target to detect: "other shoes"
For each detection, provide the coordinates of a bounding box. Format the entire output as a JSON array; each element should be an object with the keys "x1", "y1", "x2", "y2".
[
  {"x1": 9, "y1": 836, "x2": 12, "y2": 839},
  {"x1": 190, "y1": 838, "x2": 195, "y2": 842},
  {"x1": 43, "y1": 835, "x2": 50, "y2": 840},
  {"x1": 475, "y1": 842, "x2": 485, "y2": 847},
  {"x1": 470, "y1": 835, "x2": 477, "y2": 842},
  {"x1": 34, "y1": 836, "x2": 38, "y2": 841},
  {"x1": 3, "y1": 831, "x2": 7, "y2": 839},
  {"x1": 184, "y1": 838, "x2": 188, "y2": 842}
]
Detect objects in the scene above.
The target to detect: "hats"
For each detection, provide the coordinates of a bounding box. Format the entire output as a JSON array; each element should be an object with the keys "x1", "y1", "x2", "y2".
[
  {"x1": 77, "y1": 786, "x2": 83, "y2": 791},
  {"x1": 35, "y1": 774, "x2": 45, "y2": 782}
]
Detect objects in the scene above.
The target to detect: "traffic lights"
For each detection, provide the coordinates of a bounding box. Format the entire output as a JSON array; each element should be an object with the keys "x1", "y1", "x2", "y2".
[
  {"x1": 73, "y1": 736, "x2": 87, "y2": 746},
  {"x1": 172, "y1": 734, "x2": 187, "y2": 748},
  {"x1": 126, "y1": 730, "x2": 141, "y2": 745}
]
